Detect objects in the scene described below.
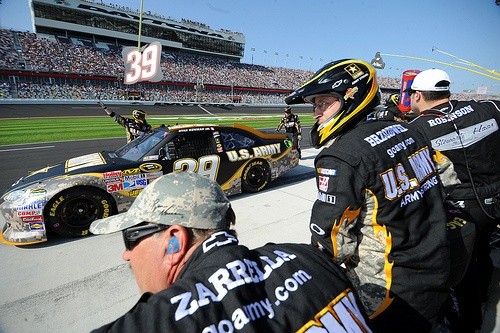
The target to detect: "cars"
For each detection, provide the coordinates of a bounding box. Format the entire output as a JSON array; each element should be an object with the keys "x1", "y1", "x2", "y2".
[{"x1": 0, "y1": 122, "x2": 301, "y2": 247}]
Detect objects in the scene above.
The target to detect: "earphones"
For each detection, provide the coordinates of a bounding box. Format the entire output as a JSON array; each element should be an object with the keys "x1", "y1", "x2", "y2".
[{"x1": 168, "y1": 236, "x2": 179, "y2": 253}]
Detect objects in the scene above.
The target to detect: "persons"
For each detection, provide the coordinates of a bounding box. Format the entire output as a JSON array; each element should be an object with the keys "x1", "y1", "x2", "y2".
[
  {"x1": 284, "y1": 58, "x2": 467, "y2": 333},
  {"x1": 449, "y1": 94, "x2": 500, "y2": 101},
  {"x1": 97, "y1": 101, "x2": 153, "y2": 144},
  {"x1": 378, "y1": 77, "x2": 402, "y2": 101},
  {"x1": 0, "y1": 0, "x2": 315, "y2": 104},
  {"x1": 376, "y1": 93, "x2": 417, "y2": 123},
  {"x1": 89, "y1": 172, "x2": 378, "y2": 333},
  {"x1": 404, "y1": 67, "x2": 500, "y2": 333},
  {"x1": 275, "y1": 106, "x2": 302, "y2": 159}
]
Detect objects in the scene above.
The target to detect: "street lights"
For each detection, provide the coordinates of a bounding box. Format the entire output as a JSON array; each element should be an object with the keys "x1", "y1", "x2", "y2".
[{"x1": 251, "y1": 48, "x2": 323, "y2": 71}]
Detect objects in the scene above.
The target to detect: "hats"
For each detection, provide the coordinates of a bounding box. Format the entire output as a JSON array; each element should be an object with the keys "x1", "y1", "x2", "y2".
[
  {"x1": 89, "y1": 170, "x2": 231, "y2": 234},
  {"x1": 403, "y1": 68, "x2": 451, "y2": 92}
]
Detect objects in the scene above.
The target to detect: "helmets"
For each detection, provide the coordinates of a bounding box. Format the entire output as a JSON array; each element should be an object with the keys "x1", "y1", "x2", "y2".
[
  {"x1": 284, "y1": 106, "x2": 291, "y2": 114},
  {"x1": 132, "y1": 109, "x2": 145, "y2": 123},
  {"x1": 285, "y1": 59, "x2": 381, "y2": 149}
]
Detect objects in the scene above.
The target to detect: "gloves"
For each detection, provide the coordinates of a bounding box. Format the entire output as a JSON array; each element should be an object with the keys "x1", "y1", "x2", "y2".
[{"x1": 99, "y1": 101, "x2": 107, "y2": 110}]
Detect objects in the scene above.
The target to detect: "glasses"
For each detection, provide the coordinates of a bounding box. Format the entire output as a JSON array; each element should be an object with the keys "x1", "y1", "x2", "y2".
[{"x1": 122, "y1": 224, "x2": 169, "y2": 250}]
